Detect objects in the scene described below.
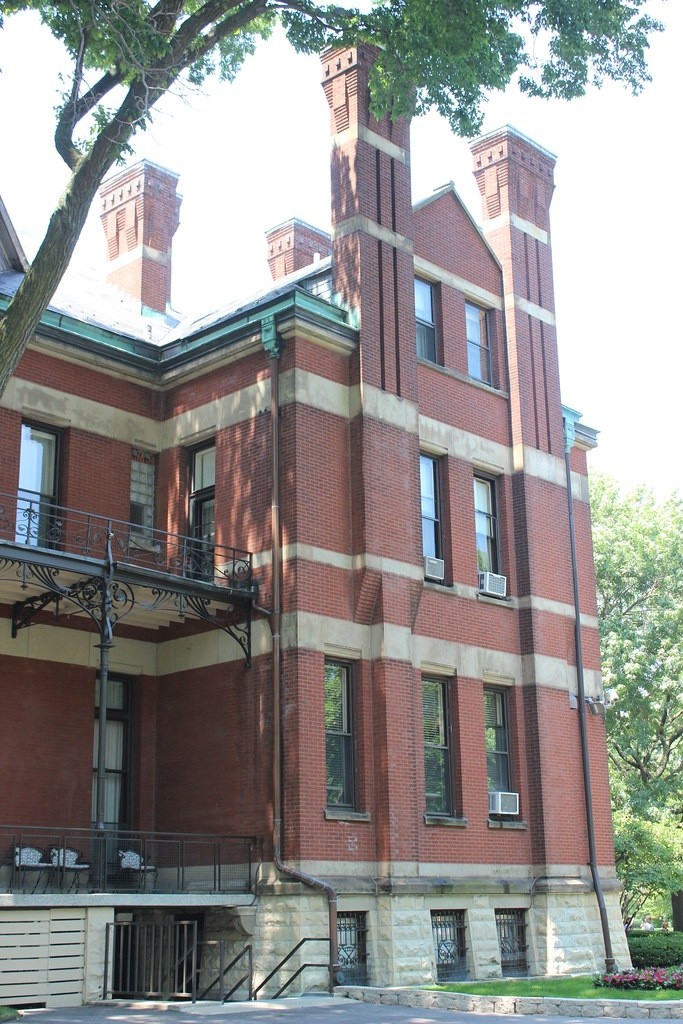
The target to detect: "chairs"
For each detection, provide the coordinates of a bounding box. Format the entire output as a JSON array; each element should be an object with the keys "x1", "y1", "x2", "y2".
[
  {"x1": 43, "y1": 844, "x2": 92, "y2": 894},
  {"x1": 6, "y1": 843, "x2": 55, "y2": 894},
  {"x1": 111, "y1": 847, "x2": 158, "y2": 894}
]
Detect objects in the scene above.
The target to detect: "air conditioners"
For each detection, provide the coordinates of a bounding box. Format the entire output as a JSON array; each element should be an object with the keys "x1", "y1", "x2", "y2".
[
  {"x1": 488, "y1": 790, "x2": 520, "y2": 816},
  {"x1": 478, "y1": 570, "x2": 507, "y2": 600},
  {"x1": 423, "y1": 557, "x2": 446, "y2": 582}
]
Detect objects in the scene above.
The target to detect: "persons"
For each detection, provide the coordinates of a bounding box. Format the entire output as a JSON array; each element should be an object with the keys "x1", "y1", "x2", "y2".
[
  {"x1": 640, "y1": 915, "x2": 654, "y2": 931},
  {"x1": 623, "y1": 917, "x2": 632, "y2": 930},
  {"x1": 662, "y1": 922, "x2": 669, "y2": 932}
]
